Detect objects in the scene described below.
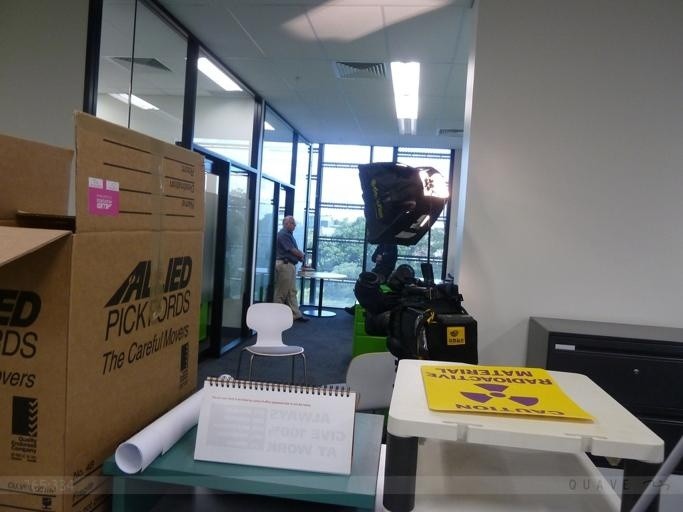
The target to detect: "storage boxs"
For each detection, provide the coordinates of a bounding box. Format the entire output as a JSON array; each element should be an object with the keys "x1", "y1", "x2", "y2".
[{"x1": 0, "y1": 107, "x2": 210, "y2": 509}]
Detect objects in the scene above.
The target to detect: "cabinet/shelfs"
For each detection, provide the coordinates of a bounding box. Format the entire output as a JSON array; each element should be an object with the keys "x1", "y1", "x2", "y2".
[{"x1": 523, "y1": 313, "x2": 683, "y2": 512}]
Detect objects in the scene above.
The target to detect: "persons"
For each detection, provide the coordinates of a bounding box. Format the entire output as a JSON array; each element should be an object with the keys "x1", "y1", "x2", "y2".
[{"x1": 272, "y1": 215, "x2": 313, "y2": 322}]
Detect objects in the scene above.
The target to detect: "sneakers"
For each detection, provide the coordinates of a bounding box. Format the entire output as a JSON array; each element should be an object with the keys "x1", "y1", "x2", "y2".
[{"x1": 344, "y1": 306, "x2": 354, "y2": 316}]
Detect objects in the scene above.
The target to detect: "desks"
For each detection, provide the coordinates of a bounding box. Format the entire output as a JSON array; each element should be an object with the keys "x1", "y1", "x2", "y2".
[{"x1": 298, "y1": 271, "x2": 347, "y2": 321}]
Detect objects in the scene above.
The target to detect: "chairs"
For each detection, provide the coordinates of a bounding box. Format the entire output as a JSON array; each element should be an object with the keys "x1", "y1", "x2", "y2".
[{"x1": 236, "y1": 303, "x2": 307, "y2": 386}]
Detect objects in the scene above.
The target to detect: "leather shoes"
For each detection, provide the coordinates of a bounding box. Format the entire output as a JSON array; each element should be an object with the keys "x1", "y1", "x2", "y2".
[{"x1": 298, "y1": 316, "x2": 312, "y2": 323}]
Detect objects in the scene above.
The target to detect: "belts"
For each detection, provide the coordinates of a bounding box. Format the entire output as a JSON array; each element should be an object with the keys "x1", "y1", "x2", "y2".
[{"x1": 276, "y1": 257, "x2": 296, "y2": 266}]
